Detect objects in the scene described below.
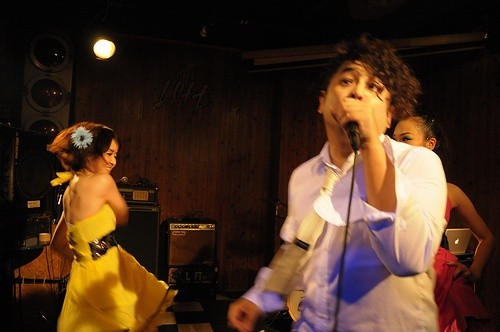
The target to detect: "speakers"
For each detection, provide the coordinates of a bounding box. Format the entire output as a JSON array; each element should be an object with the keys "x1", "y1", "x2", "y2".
[
  {"x1": 160, "y1": 223, "x2": 215, "y2": 266},
  {"x1": 112, "y1": 209, "x2": 160, "y2": 277}
]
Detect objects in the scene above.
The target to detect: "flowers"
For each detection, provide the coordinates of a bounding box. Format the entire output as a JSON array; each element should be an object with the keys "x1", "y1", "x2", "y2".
[{"x1": 70, "y1": 127, "x2": 93, "y2": 149}]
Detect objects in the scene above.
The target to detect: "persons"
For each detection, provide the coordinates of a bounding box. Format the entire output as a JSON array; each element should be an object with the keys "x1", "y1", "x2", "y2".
[
  {"x1": 227, "y1": 38, "x2": 447, "y2": 332},
  {"x1": 392, "y1": 111, "x2": 493, "y2": 332},
  {"x1": 47, "y1": 122, "x2": 178, "y2": 332}
]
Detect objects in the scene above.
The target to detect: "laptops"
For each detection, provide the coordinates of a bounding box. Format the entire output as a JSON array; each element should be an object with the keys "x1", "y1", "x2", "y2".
[{"x1": 444, "y1": 228, "x2": 471, "y2": 255}]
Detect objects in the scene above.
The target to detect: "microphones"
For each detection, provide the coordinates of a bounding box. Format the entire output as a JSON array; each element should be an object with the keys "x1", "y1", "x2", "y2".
[{"x1": 346, "y1": 121, "x2": 361, "y2": 150}]
